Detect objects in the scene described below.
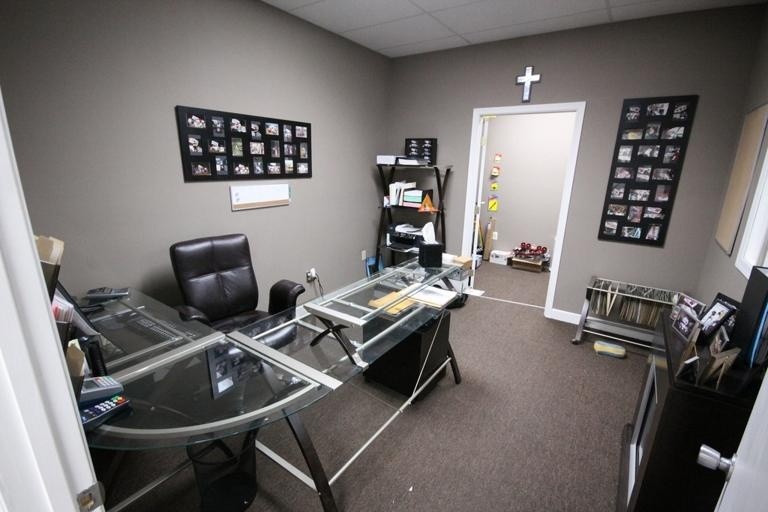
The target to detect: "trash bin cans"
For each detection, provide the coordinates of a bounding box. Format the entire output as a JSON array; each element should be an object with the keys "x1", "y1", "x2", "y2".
[{"x1": 186, "y1": 414, "x2": 257, "y2": 512}]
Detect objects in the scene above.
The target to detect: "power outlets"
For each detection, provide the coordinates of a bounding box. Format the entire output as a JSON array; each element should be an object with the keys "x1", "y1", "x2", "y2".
[{"x1": 306, "y1": 272, "x2": 315, "y2": 282}]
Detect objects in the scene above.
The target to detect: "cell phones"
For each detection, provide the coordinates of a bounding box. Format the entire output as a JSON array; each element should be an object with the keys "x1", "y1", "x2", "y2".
[{"x1": 83, "y1": 286, "x2": 129, "y2": 298}]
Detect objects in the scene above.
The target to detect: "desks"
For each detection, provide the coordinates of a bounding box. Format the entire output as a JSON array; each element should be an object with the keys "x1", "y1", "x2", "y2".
[{"x1": 67, "y1": 252, "x2": 478, "y2": 512}]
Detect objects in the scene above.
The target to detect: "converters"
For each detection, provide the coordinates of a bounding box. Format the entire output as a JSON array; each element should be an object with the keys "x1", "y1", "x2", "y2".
[{"x1": 310, "y1": 268, "x2": 316, "y2": 281}]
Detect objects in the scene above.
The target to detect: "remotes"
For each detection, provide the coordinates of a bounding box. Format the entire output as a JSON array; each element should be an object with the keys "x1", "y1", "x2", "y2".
[{"x1": 80, "y1": 395, "x2": 130, "y2": 431}]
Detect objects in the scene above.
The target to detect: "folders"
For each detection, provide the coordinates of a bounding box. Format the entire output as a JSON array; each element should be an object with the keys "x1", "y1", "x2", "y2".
[{"x1": 724, "y1": 265, "x2": 768, "y2": 380}]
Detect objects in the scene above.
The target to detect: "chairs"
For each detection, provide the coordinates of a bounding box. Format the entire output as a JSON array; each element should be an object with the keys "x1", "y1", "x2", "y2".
[{"x1": 169, "y1": 234, "x2": 305, "y2": 383}]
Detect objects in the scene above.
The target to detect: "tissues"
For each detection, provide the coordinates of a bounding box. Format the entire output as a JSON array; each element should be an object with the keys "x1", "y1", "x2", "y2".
[{"x1": 416, "y1": 221, "x2": 444, "y2": 269}]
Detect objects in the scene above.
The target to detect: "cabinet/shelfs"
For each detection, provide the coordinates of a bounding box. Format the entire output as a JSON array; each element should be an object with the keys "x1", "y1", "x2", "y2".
[
  {"x1": 373, "y1": 157, "x2": 453, "y2": 273},
  {"x1": 616, "y1": 311, "x2": 768, "y2": 511}
]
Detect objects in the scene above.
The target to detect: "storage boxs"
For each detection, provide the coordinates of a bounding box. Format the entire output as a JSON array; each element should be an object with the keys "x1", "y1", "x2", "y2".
[
  {"x1": 489, "y1": 250, "x2": 514, "y2": 266},
  {"x1": 403, "y1": 187, "x2": 433, "y2": 203},
  {"x1": 511, "y1": 256, "x2": 549, "y2": 273}
]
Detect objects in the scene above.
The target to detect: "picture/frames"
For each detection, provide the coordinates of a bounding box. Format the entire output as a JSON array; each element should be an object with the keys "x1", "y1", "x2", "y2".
[
  {"x1": 598, "y1": 94, "x2": 699, "y2": 249},
  {"x1": 698, "y1": 298, "x2": 735, "y2": 346},
  {"x1": 174, "y1": 104, "x2": 312, "y2": 183},
  {"x1": 669, "y1": 291, "x2": 706, "y2": 322},
  {"x1": 706, "y1": 291, "x2": 741, "y2": 333},
  {"x1": 672, "y1": 308, "x2": 700, "y2": 342}
]
